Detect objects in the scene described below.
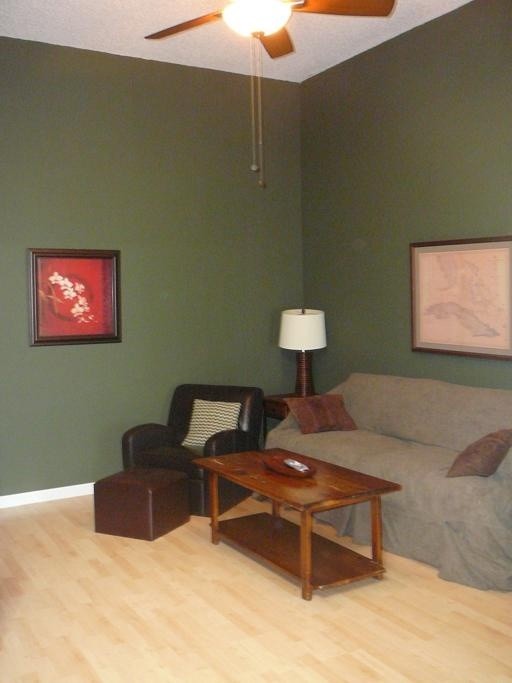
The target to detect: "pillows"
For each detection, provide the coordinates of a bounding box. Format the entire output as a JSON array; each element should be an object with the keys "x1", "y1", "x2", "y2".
[
  {"x1": 180, "y1": 397, "x2": 241, "y2": 451},
  {"x1": 284, "y1": 393, "x2": 358, "y2": 435},
  {"x1": 446, "y1": 427, "x2": 511, "y2": 478}
]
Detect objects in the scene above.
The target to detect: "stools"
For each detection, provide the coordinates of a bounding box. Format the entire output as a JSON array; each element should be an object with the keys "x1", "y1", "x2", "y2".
[{"x1": 93, "y1": 466, "x2": 189, "y2": 541}]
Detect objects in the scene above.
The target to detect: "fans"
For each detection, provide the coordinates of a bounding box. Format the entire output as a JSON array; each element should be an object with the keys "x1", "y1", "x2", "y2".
[{"x1": 143, "y1": 0, "x2": 397, "y2": 60}]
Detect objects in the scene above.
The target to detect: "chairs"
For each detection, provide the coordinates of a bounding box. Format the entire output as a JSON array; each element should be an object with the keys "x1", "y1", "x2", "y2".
[{"x1": 121, "y1": 383, "x2": 268, "y2": 517}]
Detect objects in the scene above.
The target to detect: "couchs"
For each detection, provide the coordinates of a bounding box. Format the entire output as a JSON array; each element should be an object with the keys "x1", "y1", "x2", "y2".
[{"x1": 264, "y1": 371, "x2": 511, "y2": 593}]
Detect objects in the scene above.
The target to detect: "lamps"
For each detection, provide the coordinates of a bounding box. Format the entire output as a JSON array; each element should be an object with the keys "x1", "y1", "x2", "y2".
[
  {"x1": 278, "y1": 308, "x2": 328, "y2": 397},
  {"x1": 222, "y1": 0, "x2": 294, "y2": 40}
]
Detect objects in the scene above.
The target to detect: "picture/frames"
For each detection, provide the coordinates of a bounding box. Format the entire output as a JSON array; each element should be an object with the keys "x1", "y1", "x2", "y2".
[
  {"x1": 27, "y1": 246, "x2": 124, "y2": 347},
  {"x1": 409, "y1": 234, "x2": 512, "y2": 361}
]
